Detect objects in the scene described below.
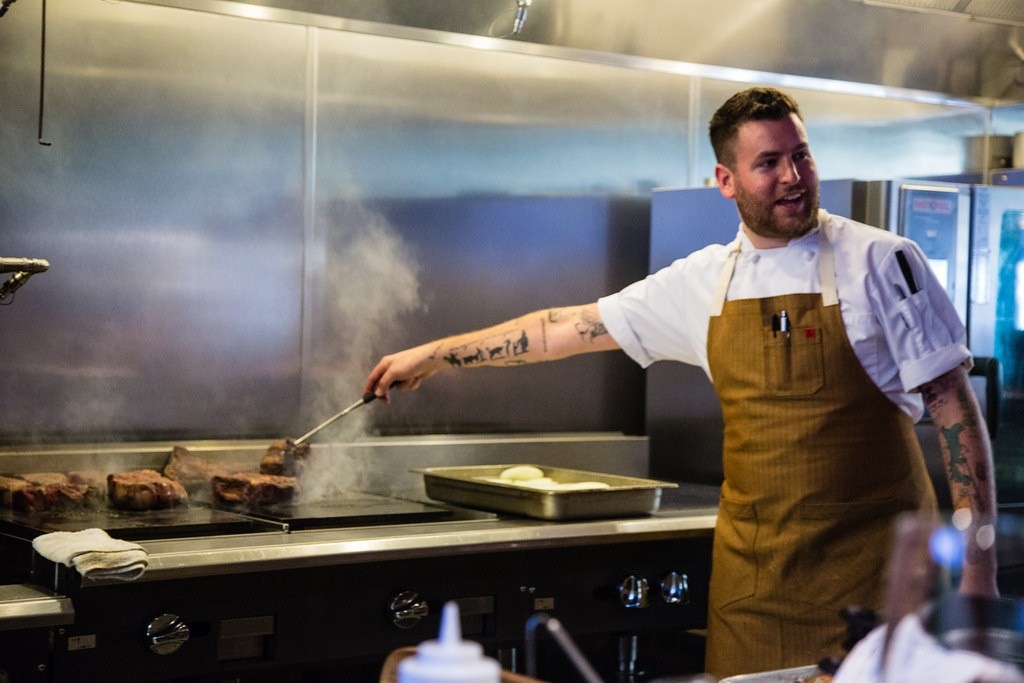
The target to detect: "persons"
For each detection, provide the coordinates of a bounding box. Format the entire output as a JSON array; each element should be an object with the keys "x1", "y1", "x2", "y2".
[{"x1": 361, "y1": 87, "x2": 1002, "y2": 683}]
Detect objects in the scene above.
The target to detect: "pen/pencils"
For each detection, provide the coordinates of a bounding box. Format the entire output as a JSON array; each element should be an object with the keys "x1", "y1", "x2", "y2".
[
  {"x1": 771, "y1": 314, "x2": 780, "y2": 338},
  {"x1": 780, "y1": 310, "x2": 787, "y2": 332}
]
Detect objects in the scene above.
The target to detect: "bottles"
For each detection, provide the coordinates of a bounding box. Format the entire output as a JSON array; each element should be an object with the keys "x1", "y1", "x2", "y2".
[{"x1": 397, "y1": 601, "x2": 501, "y2": 683}]
